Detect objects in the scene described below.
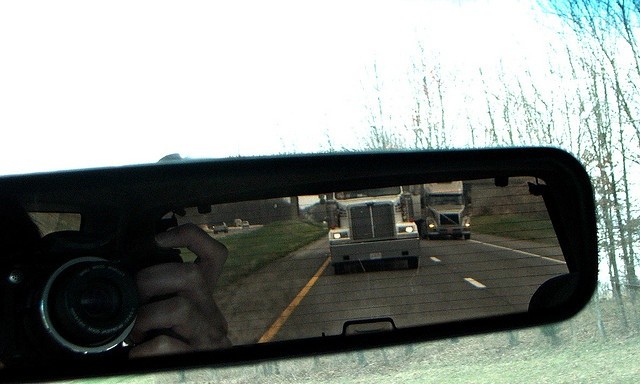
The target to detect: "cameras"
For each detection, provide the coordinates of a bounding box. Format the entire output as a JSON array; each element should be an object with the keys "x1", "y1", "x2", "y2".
[{"x1": 1, "y1": 214, "x2": 175, "y2": 357}]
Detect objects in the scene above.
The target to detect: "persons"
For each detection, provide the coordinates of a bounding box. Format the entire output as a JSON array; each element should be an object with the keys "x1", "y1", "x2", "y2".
[{"x1": 0, "y1": 173, "x2": 232, "y2": 358}]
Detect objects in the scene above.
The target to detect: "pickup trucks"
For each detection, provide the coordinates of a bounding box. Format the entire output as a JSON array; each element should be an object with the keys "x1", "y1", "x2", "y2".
[{"x1": 213, "y1": 222, "x2": 228, "y2": 234}]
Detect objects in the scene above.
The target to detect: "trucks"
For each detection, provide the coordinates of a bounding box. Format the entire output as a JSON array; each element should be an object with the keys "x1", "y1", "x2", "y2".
[
  {"x1": 318, "y1": 186, "x2": 421, "y2": 276},
  {"x1": 409, "y1": 180, "x2": 473, "y2": 240}
]
